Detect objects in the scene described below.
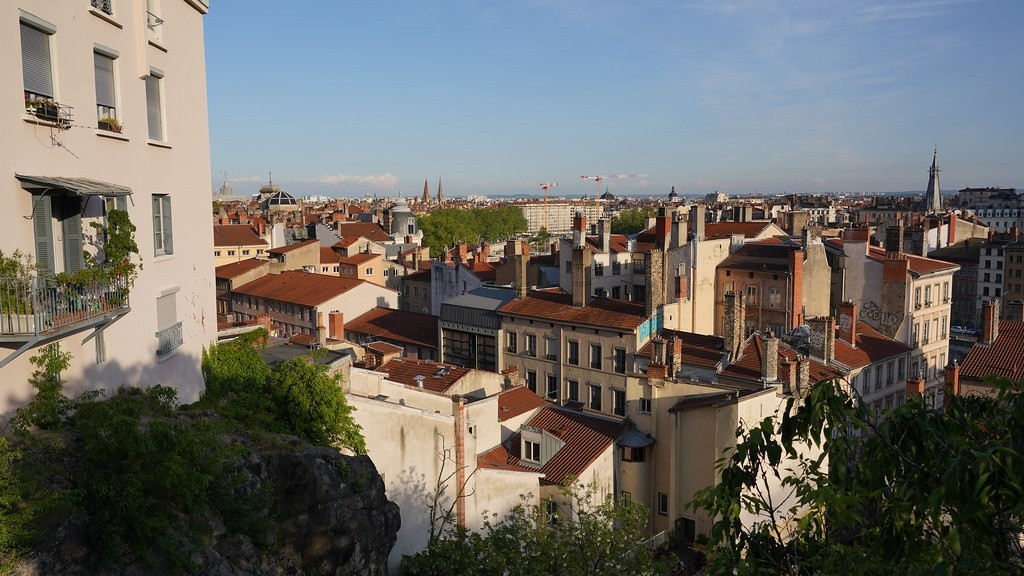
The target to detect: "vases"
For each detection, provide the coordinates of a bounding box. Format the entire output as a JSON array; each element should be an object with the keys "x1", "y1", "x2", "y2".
[{"x1": 35, "y1": 108, "x2": 56, "y2": 120}]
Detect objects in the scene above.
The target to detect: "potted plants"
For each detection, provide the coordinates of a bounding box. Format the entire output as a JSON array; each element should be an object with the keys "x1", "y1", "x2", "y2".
[
  {"x1": 31, "y1": 98, "x2": 40, "y2": 108},
  {"x1": 98, "y1": 118, "x2": 122, "y2": 133},
  {"x1": 0, "y1": 208, "x2": 143, "y2": 333}
]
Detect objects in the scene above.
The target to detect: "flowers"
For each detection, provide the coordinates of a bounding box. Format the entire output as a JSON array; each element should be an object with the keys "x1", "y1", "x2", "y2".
[{"x1": 38, "y1": 97, "x2": 55, "y2": 109}]
